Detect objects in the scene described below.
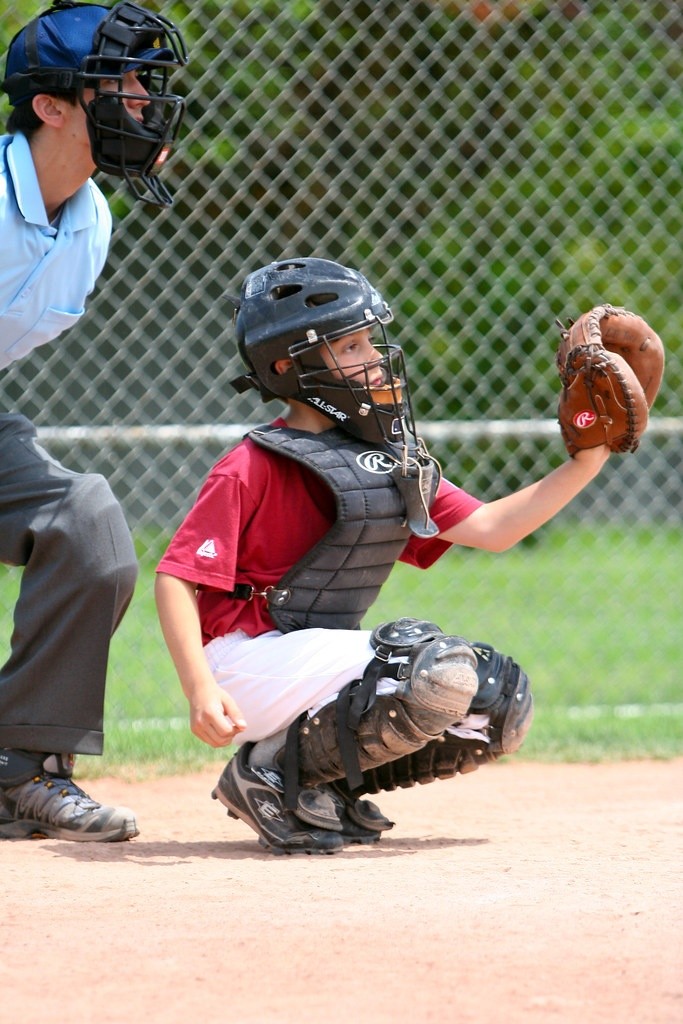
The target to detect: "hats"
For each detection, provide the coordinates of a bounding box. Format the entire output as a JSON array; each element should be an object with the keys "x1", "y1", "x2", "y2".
[{"x1": 0, "y1": 0, "x2": 178, "y2": 107}]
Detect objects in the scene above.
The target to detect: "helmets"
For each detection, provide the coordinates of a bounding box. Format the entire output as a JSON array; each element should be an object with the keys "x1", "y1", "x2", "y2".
[{"x1": 230, "y1": 256, "x2": 405, "y2": 445}]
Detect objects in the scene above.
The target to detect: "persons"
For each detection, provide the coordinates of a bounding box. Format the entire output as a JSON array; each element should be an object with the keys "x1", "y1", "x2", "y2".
[
  {"x1": 154, "y1": 258, "x2": 612, "y2": 856},
  {"x1": 0, "y1": 0, "x2": 188, "y2": 843}
]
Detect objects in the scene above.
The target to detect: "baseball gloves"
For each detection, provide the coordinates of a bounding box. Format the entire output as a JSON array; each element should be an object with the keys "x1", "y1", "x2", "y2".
[{"x1": 553, "y1": 301, "x2": 668, "y2": 462}]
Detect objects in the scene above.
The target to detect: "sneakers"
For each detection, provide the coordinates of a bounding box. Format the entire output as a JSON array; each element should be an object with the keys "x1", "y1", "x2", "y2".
[
  {"x1": 317, "y1": 782, "x2": 381, "y2": 844},
  {"x1": 0, "y1": 748, "x2": 140, "y2": 842},
  {"x1": 211, "y1": 741, "x2": 343, "y2": 855}
]
